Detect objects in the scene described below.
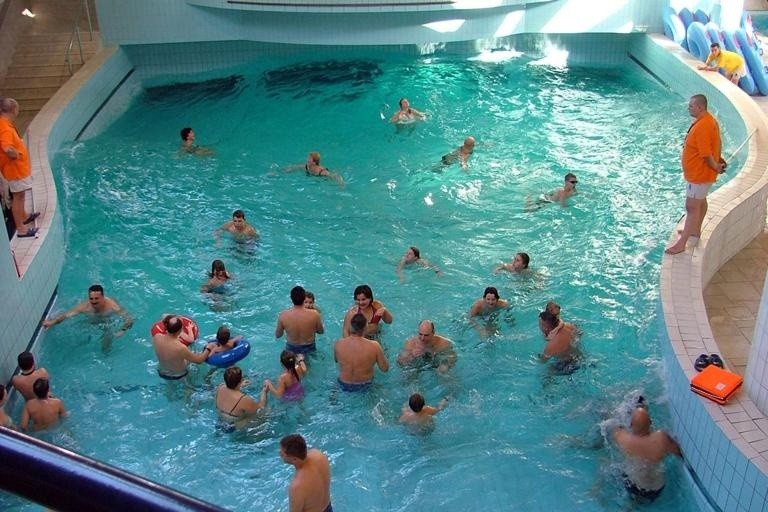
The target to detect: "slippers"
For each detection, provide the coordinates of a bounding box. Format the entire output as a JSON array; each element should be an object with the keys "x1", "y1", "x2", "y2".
[
  {"x1": 17, "y1": 227, "x2": 38, "y2": 238},
  {"x1": 695, "y1": 353, "x2": 708, "y2": 372},
  {"x1": 709, "y1": 354, "x2": 723, "y2": 368},
  {"x1": 23, "y1": 212, "x2": 40, "y2": 225}
]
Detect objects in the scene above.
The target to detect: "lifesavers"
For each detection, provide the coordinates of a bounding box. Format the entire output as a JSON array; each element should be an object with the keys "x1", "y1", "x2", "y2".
[
  {"x1": 678, "y1": 7, "x2": 695, "y2": 50},
  {"x1": 663, "y1": 7, "x2": 686, "y2": 45},
  {"x1": 722, "y1": 30, "x2": 758, "y2": 96},
  {"x1": 704, "y1": 21, "x2": 726, "y2": 77},
  {"x1": 693, "y1": 9, "x2": 708, "y2": 24},
  {"x1": 735, "y1": 24, "x2": 768, "y2": 96},
  {"x1": 687, "y1": 22, "x2": 718, "y2": 72},
  {"x1": 202, "y1": 338, "x2": 250, "y2": 367},
  {"x1": 151, "y1": 315, "x2": 198, "y2": 348}
]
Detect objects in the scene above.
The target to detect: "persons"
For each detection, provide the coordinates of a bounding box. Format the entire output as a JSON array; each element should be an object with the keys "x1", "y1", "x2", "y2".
[
  {"x1": 0, "y1": 97, "x2": 41, "y2": 237},
  {"x1": 334, "y1": 314, "x2": 390, "y2": 394},
  {"x1": 275, "y1": 286, "x2": 323, "y2": 356},
  {"x1": 213, "y1": 210, "x2": 260, "y2": 252},
  {"x1": 390, "y1": 98, "x2": 425, "y2": 123},
  {"x1": 293, "y1": 152, "x2": 343, "y2": 185},
  {"x1": 441, "y1": 136, "x2": 477, "y2": 169},
  {"x1": 399, "y1": 320, "x2": 454, "y2": 366},
  {"x1": 467, "y1": 287, "x2": 510, "y2": 338},
  {"x1": 12, "y1": 350, "x2": 52, "y2": 399},
  {"x1": 538, "y1": 312, "x2": 585, "y2": 375},
  {"x1": 267, "y1": 351, "x2": 306, "y2": 407},
  {"x1": 615, "y1": 408, "x2": 683, "y2": 459},
  {"x1": 663, "y1": 94, "x2": 728, "y2": 256},
  {"x1": 157, "y1": 313, "x2": 195, "y2": 346},
  {"x1": 397, "y1": 247, "x2": 444, "y2": 284},
  {"x1": 400, "y1": 393, "x2": 449, "y2": 426},
  {"x1": 21, "y1": 377, "x2": 68, "y2": 433},
  {"x1": 303, "y1": 291, "x2": 319, "y2": 314},
  {"x1": 343, "y1": 284, "x2": 393, "y2": 341},
  {"x1": 199, "y1": 260, "x2": 233, "y2": 296},
  {"x1": 544, "y1": 303, "x2": 562, "y2": 323},
  {"x1": 41, "y1": 284, "x2": 133, "y2": 337},
  {"x1": 206, "y1": 326, "x2": 244, "y2": 363},
  {"x1": 181, "y1": 127, "x2": 213, "y2": 157},
  {"x1": 494, "y1": 252, "x2": 541, "y2": 281},
  {"x1": 277, "y1": 433, "x2": 334, "y2": 512},
  {"x1": 154, "y1": 318, "x2": 210, "y2": 381},
  {"x1": 527, "y1": 172, "x2": 579, "y2": 209},
  {"x1": 0, "y1": 386, "x2": 18, "y2": 429},
  {"x1": 215, "y1": 367, "x2": 268, "y2": 429},
  {"x1": 698, "y1": 43, "x2": 747, "y2": 86}
]
